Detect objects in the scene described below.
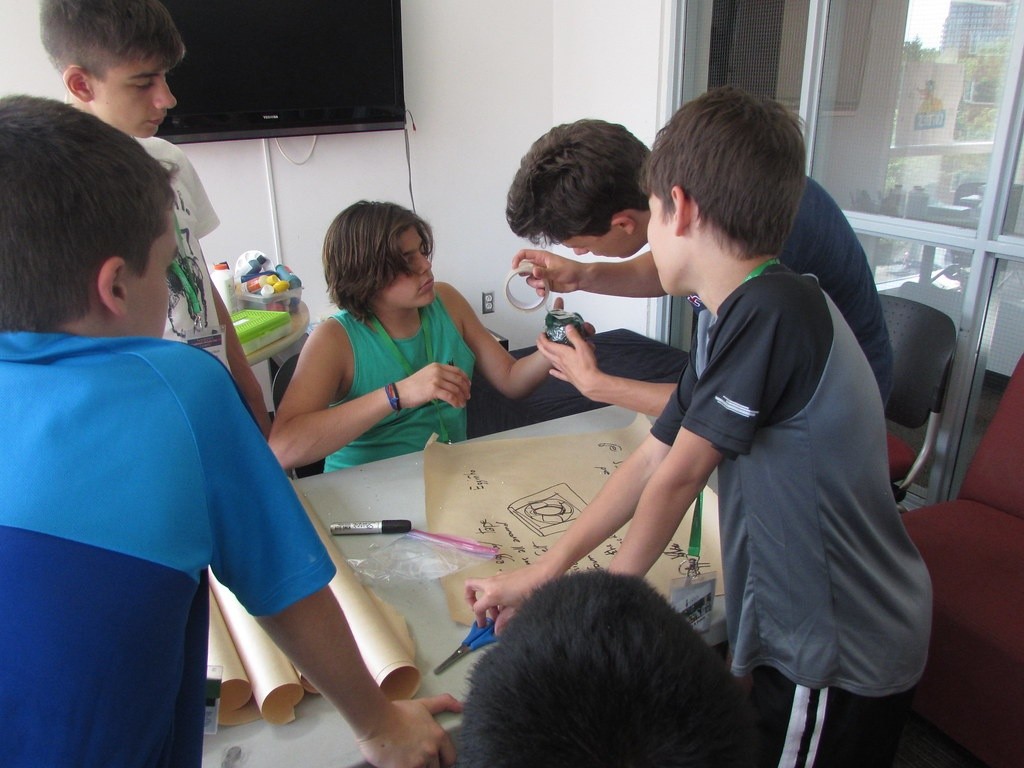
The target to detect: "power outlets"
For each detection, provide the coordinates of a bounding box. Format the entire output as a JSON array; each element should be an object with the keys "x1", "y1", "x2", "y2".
[{"x1": 481, "y1": 290, "x2": 494, "y2": 314}]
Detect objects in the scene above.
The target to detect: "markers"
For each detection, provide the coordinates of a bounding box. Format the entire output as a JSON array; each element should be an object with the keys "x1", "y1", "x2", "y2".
[{"x1": 329, "y1": 520, "x2": 412, "y2": 535}]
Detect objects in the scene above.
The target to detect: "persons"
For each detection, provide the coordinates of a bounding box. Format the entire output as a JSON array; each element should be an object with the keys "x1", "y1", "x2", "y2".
[
  {"x1": 506, "y1": 119, "x2": 895, "y2": 416},
  {"x1": 464, "y1": 84, "x2": 933, "y2": 768},
  {"x1": 40, "y1": 0, "x2": 271, "y2": 445},
  {"x1": 457, "y1": 568, "x2": 761, "y2": 768},
  {"x1": 267, "y1": 201, "x2": 595, "y2": 476},
  {"x1": 0, "y1": 96, "x2": 463, "y2": 768}
]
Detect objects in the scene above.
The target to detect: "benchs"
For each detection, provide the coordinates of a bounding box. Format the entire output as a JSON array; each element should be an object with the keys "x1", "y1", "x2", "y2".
[
  {"x1": 467, "y1": 329, "x2": 690, "y2": 437},
  {"x1": 899, "y1": 351, "x2": 1024, "y2": 768}
]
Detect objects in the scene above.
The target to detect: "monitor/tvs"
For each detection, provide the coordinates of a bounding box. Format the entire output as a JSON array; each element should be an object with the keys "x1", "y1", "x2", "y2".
[{"x1": 153, "y1": 0, "x2": 406, "y2": 144}]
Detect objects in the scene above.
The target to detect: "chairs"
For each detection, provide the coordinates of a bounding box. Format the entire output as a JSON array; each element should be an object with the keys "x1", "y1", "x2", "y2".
[
  {"x1": 271, "y1": 353, "x2": 322, "y2": 479},
  {"x1": 879, "y1": 293, "x2": 956, "y2": 501}
]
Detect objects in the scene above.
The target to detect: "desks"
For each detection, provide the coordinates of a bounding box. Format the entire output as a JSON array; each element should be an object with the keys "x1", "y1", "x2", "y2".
[
  {"x1": 202, "y1": 412, "x2": 764, "y2": 768},
  {"x1": 268, "y1": 322, "x2": 508, "y2": 387},
  {"x1": 246, "y1": 301, "x2": 310, "y2": 366}
]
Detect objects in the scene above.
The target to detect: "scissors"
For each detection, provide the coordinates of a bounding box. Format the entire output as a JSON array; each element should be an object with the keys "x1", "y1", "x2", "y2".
[{"x1": 433, "y1": 617, "x2": 500, "y2": 675}]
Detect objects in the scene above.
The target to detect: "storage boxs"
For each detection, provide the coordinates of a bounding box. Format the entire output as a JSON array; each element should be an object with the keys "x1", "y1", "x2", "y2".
[
  {"x1": 236, "y1": 287, "x2": 302, "y2": 314},
  {"x1": 230, "y1": 309, "x2": 293, "y2": 353}
]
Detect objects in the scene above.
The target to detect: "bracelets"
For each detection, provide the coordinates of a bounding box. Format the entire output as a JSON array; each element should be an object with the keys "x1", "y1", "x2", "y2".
[{"x1": 385, "y1": 382, "x2": 402, "y2": 415}]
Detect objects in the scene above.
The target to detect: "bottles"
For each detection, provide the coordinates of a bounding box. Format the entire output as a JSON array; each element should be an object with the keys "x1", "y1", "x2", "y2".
[
  {"x1": 211, "y1": 261, "x2": 238, "y2": 315},
  {"x1": 239, "y1": 255, "x2": 301, "y2": 295}
]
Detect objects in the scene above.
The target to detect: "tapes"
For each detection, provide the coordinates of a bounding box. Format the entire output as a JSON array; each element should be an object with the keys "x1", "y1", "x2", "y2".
[{"x1": 503, "y1": 264, "x2": 550, "y2": 313}]
